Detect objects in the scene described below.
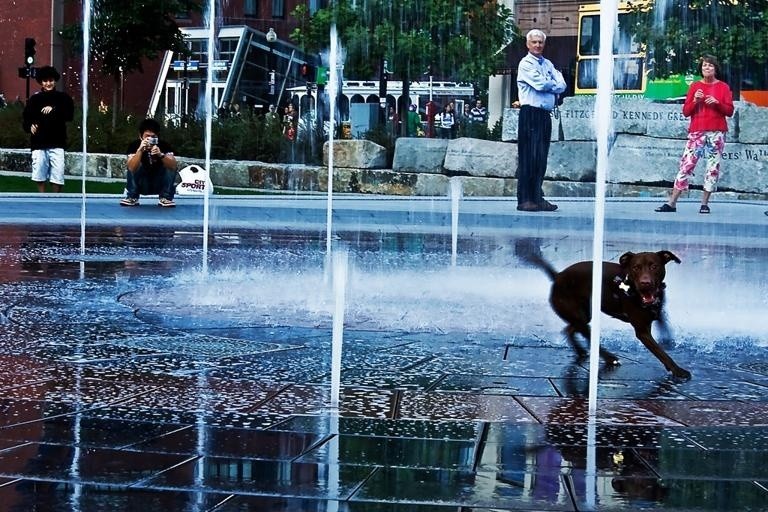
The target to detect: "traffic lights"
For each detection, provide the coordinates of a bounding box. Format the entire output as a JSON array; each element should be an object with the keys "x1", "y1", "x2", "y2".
[
  {"x1": 300, "y1": 63, "x2": 314, "y2": 81},
  {"x1": 24, "y1": 36, "x2": 36, "y2": 66}
]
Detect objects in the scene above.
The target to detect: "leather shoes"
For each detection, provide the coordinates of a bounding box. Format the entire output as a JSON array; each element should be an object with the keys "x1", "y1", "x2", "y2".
[{"x1": 515, "y1": 201, "x2": 558, "y2": 212}]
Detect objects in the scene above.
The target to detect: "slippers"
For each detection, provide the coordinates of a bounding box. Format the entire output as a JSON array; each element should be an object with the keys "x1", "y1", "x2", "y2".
[
  {"x1": 653, "y1": 203, "x2": 676, "y2": 212},
  {"x1": 698, "y1": 204, "x2": 710, "y2": 214}
]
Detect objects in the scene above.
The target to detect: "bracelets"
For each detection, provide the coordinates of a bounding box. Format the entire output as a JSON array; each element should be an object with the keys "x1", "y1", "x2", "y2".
[{"x1": 160, "y1": 152, "x2": 165, "y2": 160}]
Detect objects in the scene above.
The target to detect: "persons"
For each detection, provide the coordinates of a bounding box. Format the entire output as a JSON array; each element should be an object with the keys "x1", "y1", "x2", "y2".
[
  {"x1": 388, "y1": 104, "x2": 399, "y2": 127},
  {"x1": 440, "y1": 99, "x2": 487, "y2": 139},
  {"x1": 22, "y1": 64, "x2": 74, "y2": 192},
  {"x1": 654, "y1": 54, "x2": 734, "y2": 213},
  {"x1": 217, "y1": 101, "x2": 298, "y2": 141},
  {"x1": 119, "y1": 117, "x2": 177, "y2": 207},
  {"x1": 408, "y1": 104, "x2": 424, "y2": 137},
  {"x1": 516, "y1": 28, "x2": 567, "y2": 212}
]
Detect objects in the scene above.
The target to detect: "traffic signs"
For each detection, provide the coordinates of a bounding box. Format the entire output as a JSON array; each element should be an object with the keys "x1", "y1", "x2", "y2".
[
  {"x1": 212, "y1": 59, "x2": 228, "y2": 71},
  {"x1": 172, "y1": 60, "x2": 199, "y2": 71}
]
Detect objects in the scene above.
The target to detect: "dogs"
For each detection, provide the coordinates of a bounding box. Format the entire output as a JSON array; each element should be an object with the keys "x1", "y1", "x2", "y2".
[{"x1": 516, "y1": 240, "x2": 693, "y2": 383}]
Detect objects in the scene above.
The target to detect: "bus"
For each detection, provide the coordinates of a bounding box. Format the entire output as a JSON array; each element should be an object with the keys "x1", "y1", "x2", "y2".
[{"x1": 570, "y1": 0, "x2": 767, "y2": 104}]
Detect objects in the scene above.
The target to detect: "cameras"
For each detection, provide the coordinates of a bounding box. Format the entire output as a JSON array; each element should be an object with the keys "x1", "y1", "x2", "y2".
[{"x1": 147, "y1": 137, "x2": 158, "y2": 146}]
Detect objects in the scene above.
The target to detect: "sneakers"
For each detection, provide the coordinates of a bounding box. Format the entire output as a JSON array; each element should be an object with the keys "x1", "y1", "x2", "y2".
[
  {"x1": 158, "y1": 196, "x2": 175, "y2": 207},
  {"x1": 120, "y1": 196, "x2": 140, "y2": 207}
]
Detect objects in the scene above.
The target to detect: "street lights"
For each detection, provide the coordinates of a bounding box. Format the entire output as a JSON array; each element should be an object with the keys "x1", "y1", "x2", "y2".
[
  {"x1": 119, "y1": 65, "x2": 124, "y2": 113},
  {"x1": 264, "y1": 24, "x2": 278, "y2": 114}
]
